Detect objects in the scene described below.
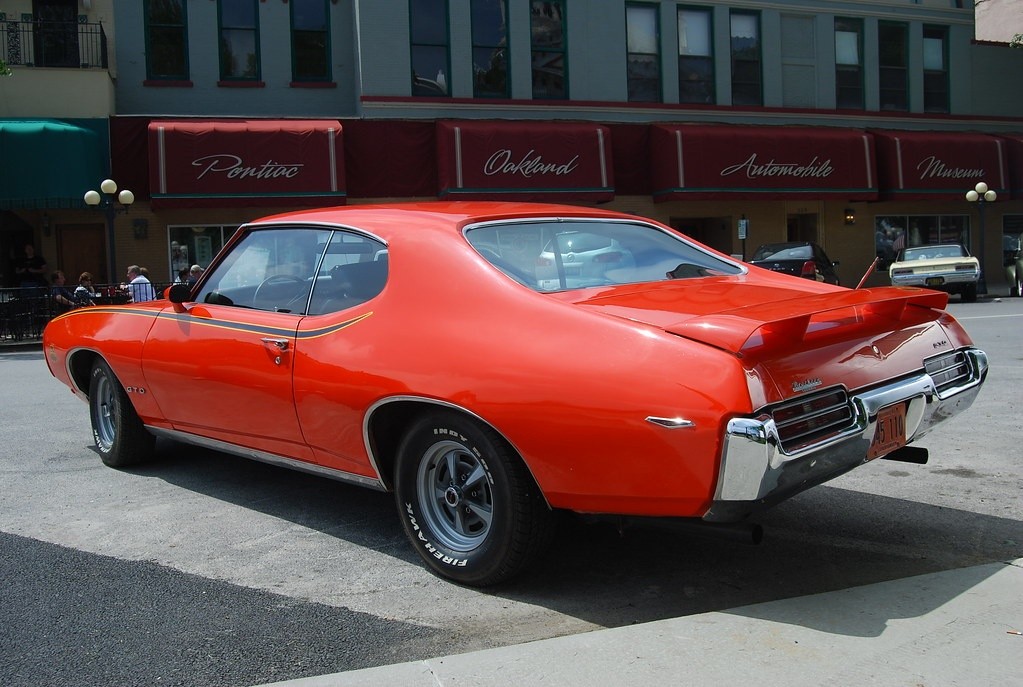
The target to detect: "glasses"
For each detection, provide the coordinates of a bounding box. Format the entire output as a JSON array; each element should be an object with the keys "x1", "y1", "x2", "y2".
[{"x1": 85, "y1": 278, "x2": 92, "y2": 282}]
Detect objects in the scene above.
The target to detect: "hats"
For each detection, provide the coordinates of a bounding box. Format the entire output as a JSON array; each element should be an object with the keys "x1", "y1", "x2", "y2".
[{"x1": 190, "y1": 264, "x2": 205, "y2": 272}]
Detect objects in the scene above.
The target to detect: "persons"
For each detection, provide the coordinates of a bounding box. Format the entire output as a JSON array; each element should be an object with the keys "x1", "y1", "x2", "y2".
[{"x1": 16, "y1": 244, "x2": 204, "y2": 325}]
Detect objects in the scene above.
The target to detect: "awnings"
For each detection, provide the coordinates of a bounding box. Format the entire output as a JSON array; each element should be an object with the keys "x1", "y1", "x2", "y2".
[
  {"x1": 0, "y1": 117, "x2": 115, "y2": 212},
  {"x1": 149, "y1": 119, "x2": 1023, "y2": 204}
]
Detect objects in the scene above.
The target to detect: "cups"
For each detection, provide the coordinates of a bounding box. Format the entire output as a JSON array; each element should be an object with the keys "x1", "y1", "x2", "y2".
[
  {"x1": 1, "y1": 292, "x2": 15, "y2": 303},
  {"x1": 101, "y1": 285, "x2": 115, "y2": 298}
]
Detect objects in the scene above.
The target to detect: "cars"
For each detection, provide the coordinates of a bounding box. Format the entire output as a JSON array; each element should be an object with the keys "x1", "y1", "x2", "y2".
[
  {"x1": 42, "y1": 203, "x2": 990, "y2": 589},
  {"x1": 535, "y1": 231, "x2": 635, "y2": 291},
  {"x1": 1005, "y1": 231, "x2": 1023, "y2": 296},
  {"x1": 889, "y1": 240, "x2": 980, "y2": 301},
  {"x1": 744, "y1": 240, "x2": 841, "y2": 287}
]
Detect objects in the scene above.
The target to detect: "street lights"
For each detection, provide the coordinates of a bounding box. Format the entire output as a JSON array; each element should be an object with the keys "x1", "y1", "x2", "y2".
[
  {"x1": 966, "y1": 182, "x2": 997, "y2": 294},
  {"x1": 84, "y1": 179, "x2": 135, "y2": 284}
]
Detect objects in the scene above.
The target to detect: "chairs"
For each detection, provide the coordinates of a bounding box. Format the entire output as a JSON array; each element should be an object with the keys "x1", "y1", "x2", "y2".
[
  {"x1": 0, "y1": 294, "x2": 163, "y2": 341},
  {"x1": 319, "y1": 260, "x2": 390, "y2": 317}
]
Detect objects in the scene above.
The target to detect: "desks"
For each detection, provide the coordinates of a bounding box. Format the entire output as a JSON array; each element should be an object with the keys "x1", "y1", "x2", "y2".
[{"x1": 88, "y1": 295, "x2": 132, "y2": 304}]
